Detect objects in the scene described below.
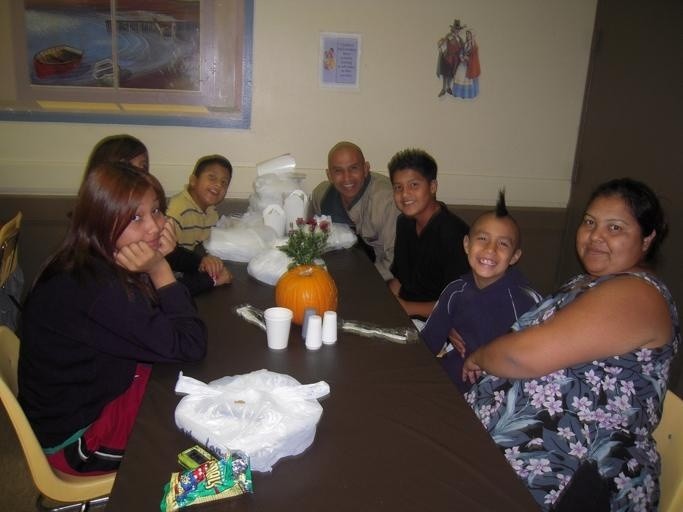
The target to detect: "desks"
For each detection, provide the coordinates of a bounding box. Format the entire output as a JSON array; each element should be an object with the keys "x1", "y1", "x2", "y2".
[{"x1": 102, "y1": 242, "x2": 545, "y2": 512}]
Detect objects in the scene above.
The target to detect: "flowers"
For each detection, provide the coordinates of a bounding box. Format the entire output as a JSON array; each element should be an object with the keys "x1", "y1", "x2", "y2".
[{"x1": 276, "y1": 215, "x2": 330, "y2": 273}]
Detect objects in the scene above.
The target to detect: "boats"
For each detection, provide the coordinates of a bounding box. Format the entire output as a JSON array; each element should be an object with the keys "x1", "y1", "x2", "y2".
[
  {"x1": 93, "y1": 58, "x2": 121, "y2": 85},
  {"x1": 32, "y1": 44, "x2": 85, "y2": 81}
]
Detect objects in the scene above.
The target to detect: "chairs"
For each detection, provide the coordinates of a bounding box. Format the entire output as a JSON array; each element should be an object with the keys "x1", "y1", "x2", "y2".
[
  {"x1": 0, "y1": 211, "x2": 117, "y2": 511},
  {"x1": 651, "y1": 389, "x2": 683, "y2": 511}
]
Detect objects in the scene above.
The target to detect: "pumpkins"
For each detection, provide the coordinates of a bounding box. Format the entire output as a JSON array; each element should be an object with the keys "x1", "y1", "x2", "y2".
[{"x1": 274, "y1": 265, "x2": 338, "y2": 326}]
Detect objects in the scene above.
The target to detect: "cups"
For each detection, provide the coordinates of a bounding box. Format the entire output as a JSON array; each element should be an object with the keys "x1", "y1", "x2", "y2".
[{"x1": 264, "y1": 307, "x2": 339, "y2": 352}]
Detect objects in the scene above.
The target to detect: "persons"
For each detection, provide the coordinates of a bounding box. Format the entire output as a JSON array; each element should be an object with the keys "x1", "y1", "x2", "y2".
[
  {"x1": 313, "y1": 138, "x2": 402, "y2": 283},
  {"x1": 462, "y1": 175, "x2": 679, "y2": 512},
  {"x1": 418, "y1": 186, "x2": 543, "y2": 397},
  {"x1": 19, "y1": 157, "x2": 208, "y2": 475},
  {"x1": 166, "y1": 153, "x2": 231, "y2": 252},
  {"x1": 82, "y1": 128, "x2": 231, "y2": 297},
  {"x1": 385, "y1": 147, "x2": 471, "y2": 334}
]
